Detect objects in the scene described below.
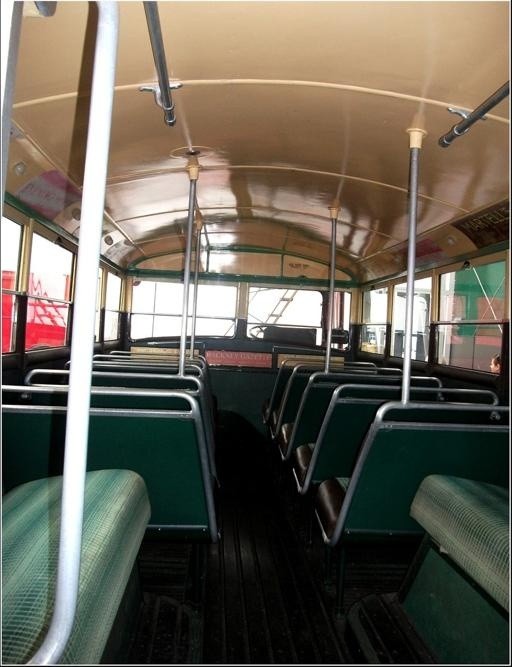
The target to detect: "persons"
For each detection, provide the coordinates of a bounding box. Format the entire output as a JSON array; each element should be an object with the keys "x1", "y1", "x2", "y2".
[{"x1": 488, "y1": 352, "x2": 501, "y2": 373}]
